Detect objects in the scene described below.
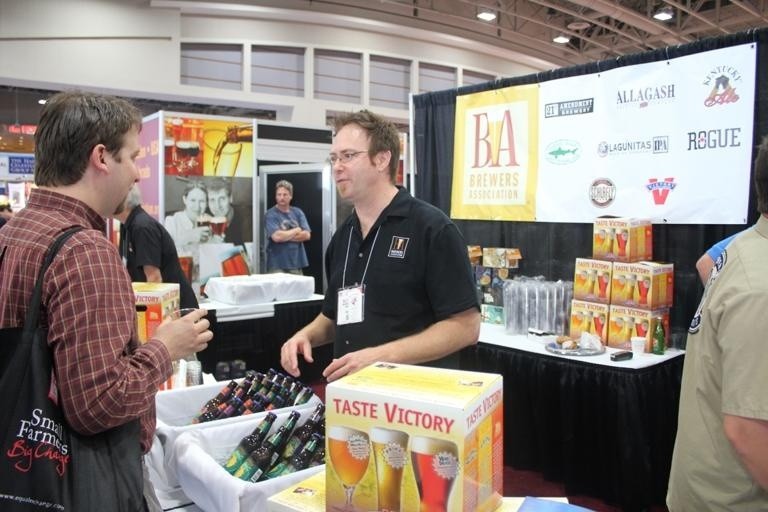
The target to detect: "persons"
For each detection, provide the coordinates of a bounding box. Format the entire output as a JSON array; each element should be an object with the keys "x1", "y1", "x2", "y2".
[
  {"x1": 0, "y1": 203, "x2": 13, "y2": 221},
  {"x1": 0, "y1": 90, "x2": 215, "y2": 512},
  {"x1": 113, "y1": 184, "x2": 199, "y2": 316},
  {"x1": 165, "y1": 180, "x2": 211, "y2": 265},
  {"x1": 695, "y1": 233, "x2": 737, "y2": 290},
  {"x1": 666, "y1": 136, "x2": 768, "y2": 512},
  {"x1": 264, "y1": 180, "x2": 312, "y2": 276},
  {"x1": 280, "y1": 109, "x2": 481, "y2": 383},
  {"x1": 207, "y1": 177, "x2": 253, "y2": 245}
]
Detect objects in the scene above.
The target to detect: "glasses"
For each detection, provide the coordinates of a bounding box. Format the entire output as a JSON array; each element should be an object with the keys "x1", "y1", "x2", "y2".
[{"x1": 324, "y1": 150, "x2": 370, "y2": 165}]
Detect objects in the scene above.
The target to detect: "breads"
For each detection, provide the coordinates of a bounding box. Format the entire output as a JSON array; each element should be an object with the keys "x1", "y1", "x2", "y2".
[{"x1": 556, "y1": 335, "x2": 577, "y2": 350}]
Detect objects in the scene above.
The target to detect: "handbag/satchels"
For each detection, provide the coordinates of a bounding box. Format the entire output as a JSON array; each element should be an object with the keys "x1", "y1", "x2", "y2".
[{"x1": 0, "y1": 223, "x2": 148, "y2": 512}]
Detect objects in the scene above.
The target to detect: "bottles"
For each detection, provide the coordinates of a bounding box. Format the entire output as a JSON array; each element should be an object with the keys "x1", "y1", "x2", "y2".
[
  {"x1": 652, "y1": 317, "x2": 665, "y2": 353},
  {"x1": 195, "y1": 368, "x2": 315, "y2": 425},
  {"x1": 227, "y1": 403, "x2": 325, "y2": 484},
  {"x1": 225, "y1": 124, "x2": 254, "y2": 144},
  {"x1": 501, "y1": 274, "x2": 575, "y2": 335}
]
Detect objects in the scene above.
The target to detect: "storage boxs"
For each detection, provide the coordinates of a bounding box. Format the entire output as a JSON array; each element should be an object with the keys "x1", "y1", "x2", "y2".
[
  {"x1": 128, "y1": 279, "x2": 181, "y2": 346},
  {"x1": 461, "y1": 246, "x2": 521, "y2": 325},
  {"x1": 565, "y1": 212, "x2": 674, "y2": 354},
  {"x1": 148, "y1": 368, "x2": 322, "y2": 490},
  {"x1": 326, "y1": 359, "x2": 503, "y2": 512},
  {"x1": 171, "y1": 402, "x2": 332, "y2": 511}
]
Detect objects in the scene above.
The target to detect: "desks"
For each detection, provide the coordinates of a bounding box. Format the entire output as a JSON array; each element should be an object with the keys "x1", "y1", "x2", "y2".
[
  {"x1": 191, "y1": 294, "x2": 335, "y2": 388},
  {"x1": 459, "y1": 323, "x2": 685, "y2": 504}
]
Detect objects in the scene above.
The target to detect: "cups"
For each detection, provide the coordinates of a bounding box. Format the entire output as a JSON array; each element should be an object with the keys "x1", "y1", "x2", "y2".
[
  {"x1": 212, "y1": 217, "x2": 228, "y2": 241},
  {"x1": 201, "y1": 129, "x2": 242, "y2": 175},
  {"x1": 194, "y1": 219, "x2": 210, "y2": 242},
  {"x1": 576, "y1": 269, "x2": 651, "y2": 309},
  {"x1": 667, "y1": 330, "x2": 682, "y2": 351},
  {"x1": 161, "y1": 359, "x2": 204, "y2": 392},
  {"x1": 164, "y1": 119, "x2": 203, "y2": 175},
  {"x1": 629, "y1": 337, "x2": 647, "y2": 358},
  {"x1": 223, "y1": 254, "x2": 250, "y2": 277},
  {"x1": 595, "y1": 227, "x2": 627, "y2": 259},
  {"x1": 168, "y1": 309, "x2": 201, "y2": 356},
  {"x1": 369, "y1": 426, "x2": 410, "y2": 511},
  {"x1": 410, "y1": 433, "x2": 458, "y2": 511},
  {"x1": 573, "y1": 309, "x2": 648, "y2": 348}
]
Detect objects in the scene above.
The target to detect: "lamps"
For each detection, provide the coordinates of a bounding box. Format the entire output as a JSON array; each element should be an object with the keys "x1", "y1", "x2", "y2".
[
  {"x1": 475, "y1": 6, "x2": 496, "y2": 23},
  {"x1": 652, "y1": 1, "x2": 673, "y2": 21},
  {"x1": 552, "y1": 32, "x2": 569, "y2": 44},
  {"x1": 566, "y1": 3, "x2": 590, "y2": 30}
]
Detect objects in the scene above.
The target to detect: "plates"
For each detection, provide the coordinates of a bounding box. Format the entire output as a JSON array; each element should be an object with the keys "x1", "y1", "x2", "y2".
[{"x1": 547, "y1": 342, "x2": 607, "y2": 357}]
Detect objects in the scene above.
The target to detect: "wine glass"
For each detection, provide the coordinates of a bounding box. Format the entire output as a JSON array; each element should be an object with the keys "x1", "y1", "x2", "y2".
[{"x1": 328, "y1": 425, "x2": 368, "y2": 511}]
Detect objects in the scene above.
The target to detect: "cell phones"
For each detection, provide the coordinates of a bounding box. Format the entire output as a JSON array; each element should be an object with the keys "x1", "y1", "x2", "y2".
[{"x1": 610, "y1": 350, "x2": 633, "y2": 361}]
